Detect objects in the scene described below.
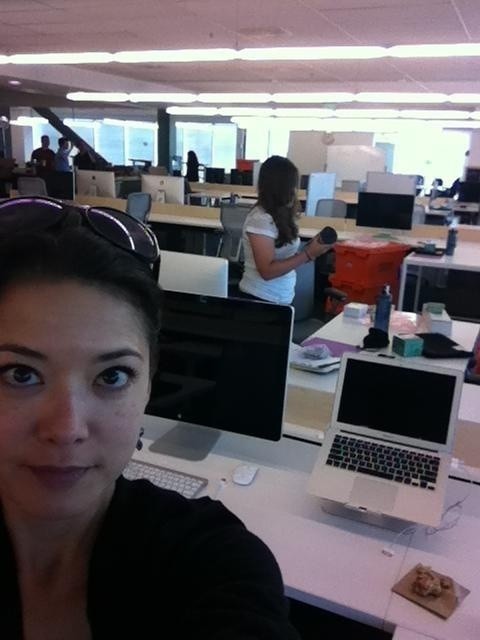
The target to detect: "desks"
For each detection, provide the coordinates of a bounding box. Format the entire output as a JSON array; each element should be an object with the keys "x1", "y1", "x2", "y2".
[
  {"x1": 110, "y1": 301, "x2": 480, "y2": 636},
  {"x1": 87, "y1": 187, "x2": 479, "y2": 298}
]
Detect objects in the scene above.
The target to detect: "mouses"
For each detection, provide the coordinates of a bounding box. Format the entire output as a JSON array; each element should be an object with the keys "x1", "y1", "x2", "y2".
[{"x1": 232, "y1": 464, "x2": 260, "y2": 486}]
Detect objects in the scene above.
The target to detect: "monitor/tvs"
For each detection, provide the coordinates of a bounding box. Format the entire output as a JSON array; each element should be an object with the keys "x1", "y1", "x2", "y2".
[
  {"x1": 76, "y1": 169, "x2": 117, "y2": 199},
  {"x1": 158, "y1": 248, "x2": 230, "y2": 299},
  {"x1": 143, "y1": 288, "x2": 295, "y2": 462},
  {"x1": 141, "y1": 173, "x2": 185, "y2": 206},
  {"x1": 355, "y1": 190, "x2": 416, "y2": 240}
]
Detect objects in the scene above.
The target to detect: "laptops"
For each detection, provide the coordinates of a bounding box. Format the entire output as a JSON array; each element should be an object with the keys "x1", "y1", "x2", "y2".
[{"x1": 307, "y1": 352, "x2": 465, "y2": 529}]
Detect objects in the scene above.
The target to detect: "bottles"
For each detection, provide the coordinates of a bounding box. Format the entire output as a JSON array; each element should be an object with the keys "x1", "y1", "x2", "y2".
[
  {"x1": 444, "y1": 226, "x2": 459, "y2": 255},
  {"x1": 374, "y1": 282, "x2": 394, "y2": 333}
]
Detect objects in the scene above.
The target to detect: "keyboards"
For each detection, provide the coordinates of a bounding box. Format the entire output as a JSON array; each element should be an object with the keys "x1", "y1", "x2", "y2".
[{"x1": 122, "y1": 459, "x2": 209, "y2": 499}]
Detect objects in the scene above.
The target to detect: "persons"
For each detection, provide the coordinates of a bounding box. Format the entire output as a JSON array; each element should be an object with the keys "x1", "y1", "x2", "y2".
[
  {"x1": 0, "y1": 194, "x2": 288, "y2": 640},
  {"x1": 184, "y1": 151, "x2": 198, "y2": 183},
  {"x1": 238, "y1": 155, "x2": 337, "y2": 307},
  {"x1": 30, "y1": 135, "x2": 97, "y2": 171}
]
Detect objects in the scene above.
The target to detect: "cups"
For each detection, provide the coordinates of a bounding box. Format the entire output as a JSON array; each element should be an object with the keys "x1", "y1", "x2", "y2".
[{"x1": 305, "y1": 226, "x2": 339, "y2": 249}]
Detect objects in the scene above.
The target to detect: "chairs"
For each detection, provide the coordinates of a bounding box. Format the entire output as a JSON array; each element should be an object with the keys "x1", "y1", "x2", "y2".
[
  {"x1": 17, "y1": 177, "x2": 48, "y2": 196},
  {"x1": 122, "y1": 192, "x2": 158, "y2": 263},
  {"x1": 130, "y1": 159, "x2": 150, "y2": 169},
  {"x1": 209, "y1": 201, "x2": 271, "y2": 289}
]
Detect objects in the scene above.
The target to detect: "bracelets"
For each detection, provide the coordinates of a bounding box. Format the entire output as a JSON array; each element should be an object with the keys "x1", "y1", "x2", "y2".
[{"x1": 302, "y1": 247, "x2": 315, "y2": 261}]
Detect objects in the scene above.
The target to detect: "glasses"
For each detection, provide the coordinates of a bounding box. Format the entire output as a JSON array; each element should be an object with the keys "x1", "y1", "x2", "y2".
[{"x1": 1, "y1": 195, "x2": 160, "y2": 277}]
